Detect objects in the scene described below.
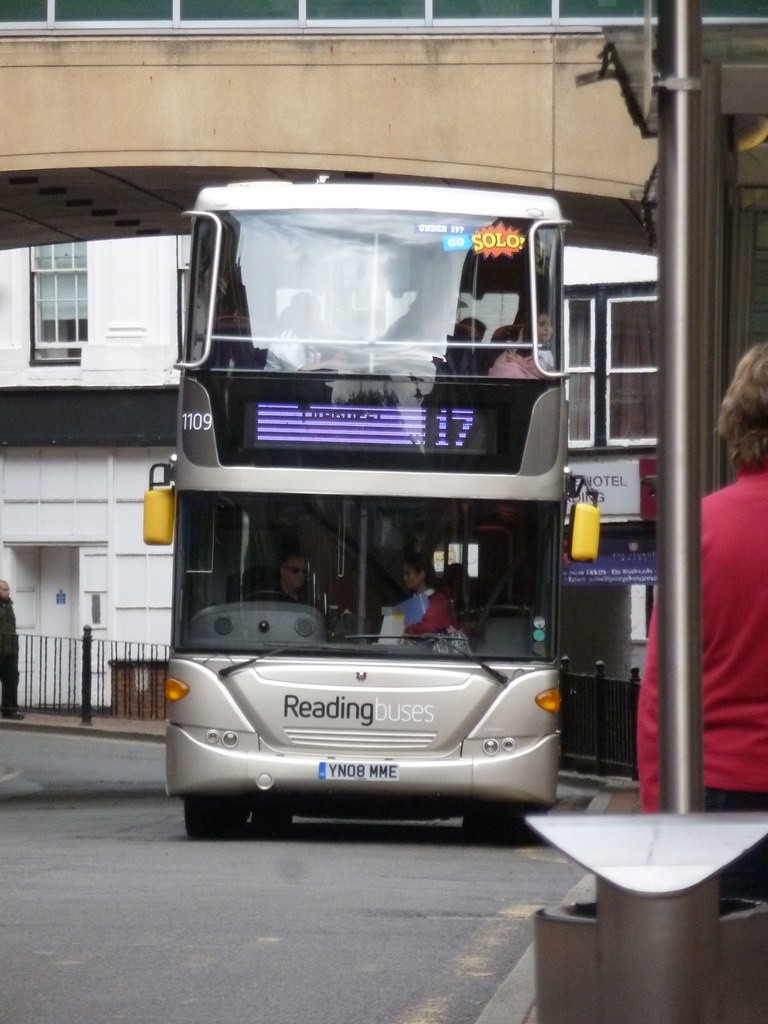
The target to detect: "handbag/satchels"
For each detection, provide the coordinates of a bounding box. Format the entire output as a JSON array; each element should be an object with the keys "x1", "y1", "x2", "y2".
[{"x1": 432, "y1": 629, "x2": 472, "y2": 655}]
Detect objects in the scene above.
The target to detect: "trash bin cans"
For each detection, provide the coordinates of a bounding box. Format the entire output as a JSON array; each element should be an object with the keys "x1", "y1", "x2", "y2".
[{"x1": 524, "y1": 809, "x2": 768, "y2": 1024}]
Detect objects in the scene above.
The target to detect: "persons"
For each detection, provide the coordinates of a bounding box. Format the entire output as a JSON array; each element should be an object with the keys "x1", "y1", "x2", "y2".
[
  {"x1": 487, "y1": 308, "x2": 554, "y2": 380},
  {"x1": 0, "y1": 580, "x2": 26, "y2": 720},
  {"x1": 265, "y1": 290, "x2": 487, "y2": 375},
  {"x1": 272, "y1": 554, "x2": 309, "y2": 603},
  {"x1": 635, "y1": 338, "x2": 768, "y2": 896},
  {"x1": 400, "y1": 554, "x2": 456, "y2": 638}
]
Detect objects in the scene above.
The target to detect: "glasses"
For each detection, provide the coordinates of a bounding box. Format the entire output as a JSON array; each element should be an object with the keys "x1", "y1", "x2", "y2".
[{"x1": 281, "y1": 565, "x2": 308, "y2": 573}]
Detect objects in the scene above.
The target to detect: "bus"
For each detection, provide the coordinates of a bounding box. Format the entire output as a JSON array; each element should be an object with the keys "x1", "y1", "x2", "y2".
[{"x1": 143, "y1": 181, "x2": 601, "y2": 843}]
